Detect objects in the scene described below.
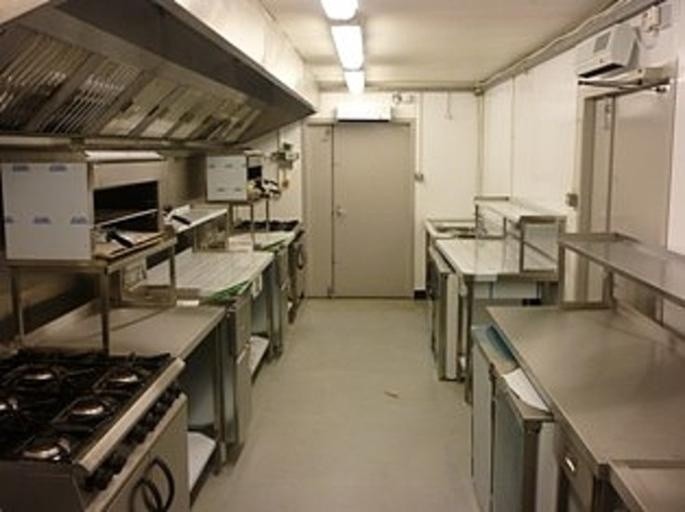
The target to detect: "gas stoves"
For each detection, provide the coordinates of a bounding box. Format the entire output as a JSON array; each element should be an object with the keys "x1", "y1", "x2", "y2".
[{"x1": 0, "y1": 343, "x2": 187, "y2": 511}]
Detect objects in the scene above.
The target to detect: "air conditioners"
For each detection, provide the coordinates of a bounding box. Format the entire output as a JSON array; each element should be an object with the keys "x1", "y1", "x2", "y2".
[{"x1": 574, "y1": 25, "x2": 638, "y2": 82}]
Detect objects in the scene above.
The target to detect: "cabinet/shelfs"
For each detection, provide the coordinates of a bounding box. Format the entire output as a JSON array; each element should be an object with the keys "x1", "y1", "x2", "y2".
[
  {"x1": 424, "y1": 197, "x2": 560, "y2": 383},
  {"x1": 24, "y1": 292, "x2": 228, "y2": 506},
  {"x1": 225, "y1": 218, "x2": 308, "y2": 324},
  {"x1": 0, "y1": 132, "x2": 180, "y2": 353},
  {"x1": 143, "y1": 247, "x2": 284, "y2": 446},
  {"x1": 469, "y1": 298, "x2": 684, "y2": 512}
]
